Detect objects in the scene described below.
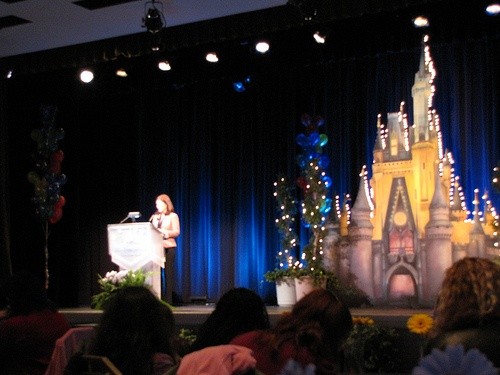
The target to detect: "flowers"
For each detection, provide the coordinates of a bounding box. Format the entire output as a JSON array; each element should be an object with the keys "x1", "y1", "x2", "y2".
[
  {"x1": 406, "y1": 314, "x2": 435, "y2": 358},
  {"x1": 346, "y1": 316, "x2": 375, "y2": 355},
  {"x1": 91, "y1": 268, "x2": 176, "y2": 312}
]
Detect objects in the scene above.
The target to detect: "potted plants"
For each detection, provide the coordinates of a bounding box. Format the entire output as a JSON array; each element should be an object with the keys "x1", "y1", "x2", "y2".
[
  {"x1": 289, "y1": 268, "x2": 334, "y2": 303},
  {"x1": 264, "y1": 267, "x2": 297, "y2": 307}
]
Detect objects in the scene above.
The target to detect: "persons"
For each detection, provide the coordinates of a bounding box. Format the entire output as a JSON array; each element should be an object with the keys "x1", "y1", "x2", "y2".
[
  {"x1": 182, "y1": 287, "x2": 270, "y2": 357},
  {"x1": 421, "y1": 257, "x2": 500, "y2": 369},
  {"x1": 149, "y1": 194, "x2": 180, "y2": 304},
  {"x1": 92, "y1": 286, "x2": 177, "y2": 375},
  {"x1": 229, "y1": 291, "x2": 353, "y2": 375}
]
